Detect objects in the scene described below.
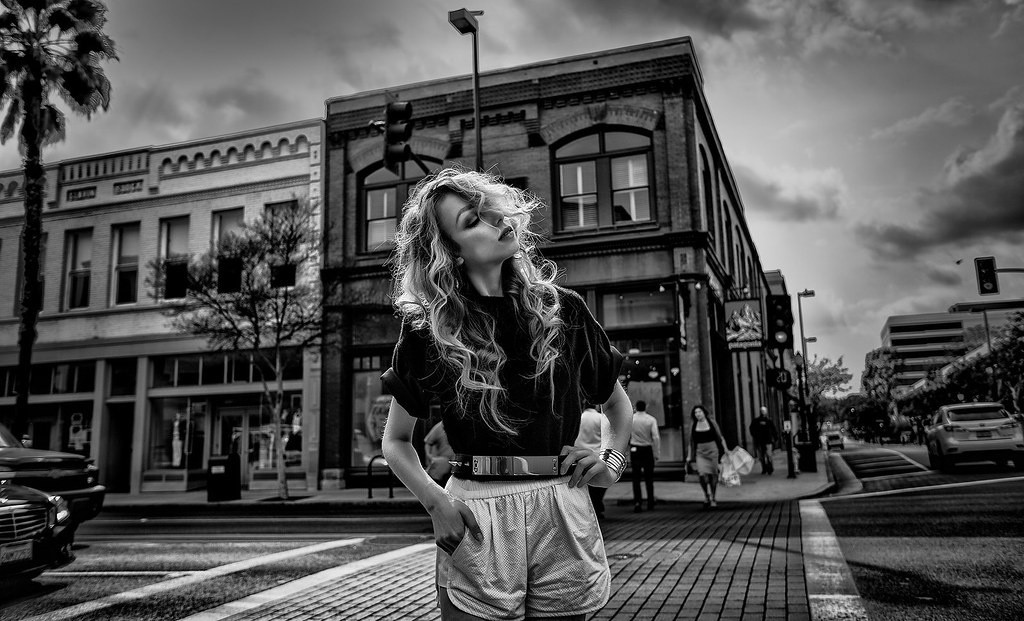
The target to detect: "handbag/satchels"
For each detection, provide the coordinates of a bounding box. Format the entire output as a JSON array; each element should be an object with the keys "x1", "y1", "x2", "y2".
[
  {"x1": 685, "y1": 456, "x2": 693, "y2": 475},
  {"x1": 729, "y1": 446, "x2": 754, "y2": 476},
  {"x1": 717, "y1": 453, "x2": 741, "y2": 488}
]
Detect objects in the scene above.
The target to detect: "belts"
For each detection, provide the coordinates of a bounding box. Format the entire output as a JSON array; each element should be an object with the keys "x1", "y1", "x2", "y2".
[{"x1": 449, "y1": 453, "x2": 577, "y2": 479}]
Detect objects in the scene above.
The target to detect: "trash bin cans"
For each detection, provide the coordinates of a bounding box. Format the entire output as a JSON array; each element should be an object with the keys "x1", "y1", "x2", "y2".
[{"x1": 207, "y1": 452, "x2": 242, "y2": 502}]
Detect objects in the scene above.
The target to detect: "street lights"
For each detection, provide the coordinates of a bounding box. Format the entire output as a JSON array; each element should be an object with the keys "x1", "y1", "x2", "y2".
[
  {"x1": 798, "y1": 290, "x2": 818, "y2": 472},
  {"x1": 447, "y1": 9, "x2": 487, "y2": 173}
]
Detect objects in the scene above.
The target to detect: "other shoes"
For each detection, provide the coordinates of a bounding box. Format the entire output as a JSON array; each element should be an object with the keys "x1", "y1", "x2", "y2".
[
  {"x1": 597, "y1": 513, "x2": 604, "y2": 519},
  {"x1": 647, "y1": 498, "x2": 656, "y2": 510},
  {"x1": 635, "y1": 503, "x2": 643, "y2": 513}
]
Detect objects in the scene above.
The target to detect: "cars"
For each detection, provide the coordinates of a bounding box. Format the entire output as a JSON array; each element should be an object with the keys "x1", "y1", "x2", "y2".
[{"x1": 2, "y1": 480, "x2": 76, "y2": 589}]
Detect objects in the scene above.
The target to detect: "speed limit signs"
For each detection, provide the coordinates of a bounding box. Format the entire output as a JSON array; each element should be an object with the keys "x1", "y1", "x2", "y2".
[{"x1": 772, "y1": 369, "x2": 792, "y2": 391}]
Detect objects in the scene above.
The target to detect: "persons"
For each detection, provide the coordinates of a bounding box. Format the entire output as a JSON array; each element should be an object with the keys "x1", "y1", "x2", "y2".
[
  {"x1": 291, "y1": 408, "x2": 301, "y2": 427},
  {"x1": 572, "y1": 398, "x2": 603, "y2": 524},
  {"x1": 168, "y1": 412, "x2": 188, "y2": 469},
  {"x1": 686, "y1": 404, "x2": 731, "y2": 508},
  {"x1": 280, "y1": 408, "x2": 291, "y2": 426},
  {"x1": 377, "y1": 166, "x2": 634, "y2": 621},
  {"x1": 776, "y1": 411, "x2": 933, "y2": 456},
  {"x1": 749, "y1": 406, "x2": 778, "y2": 475},
  {"x1": 627, "y1": 400, "x2": 662, "y2": 513}
]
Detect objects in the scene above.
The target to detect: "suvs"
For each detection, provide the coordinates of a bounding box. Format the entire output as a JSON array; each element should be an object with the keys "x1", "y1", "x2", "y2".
[
  {"x1": 924, "y1": 403, "x2": 1024, "y2": 471},
  {"x1": 825, "y1": 431, "x2": 845, "y2": 450},
  {"x1": 0, "y1": 424, "x2": 106, "y2": 540}
]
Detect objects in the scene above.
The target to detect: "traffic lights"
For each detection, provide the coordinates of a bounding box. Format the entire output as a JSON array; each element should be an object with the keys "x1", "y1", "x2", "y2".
[
  {"x1": 974, "y1": 257, "x2": 1001, "y2": 296},
  {"x1": 384, "y1": 89, "x2": 413, "y2": 173},
  {"x1": 765, "y1": 292, "x2": 796, "y2": 349}
]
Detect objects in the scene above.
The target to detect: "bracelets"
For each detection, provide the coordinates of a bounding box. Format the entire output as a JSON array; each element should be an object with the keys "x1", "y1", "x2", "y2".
[{"x1": 599, "y1": 448, "x2": 628, "y2": 483}]
